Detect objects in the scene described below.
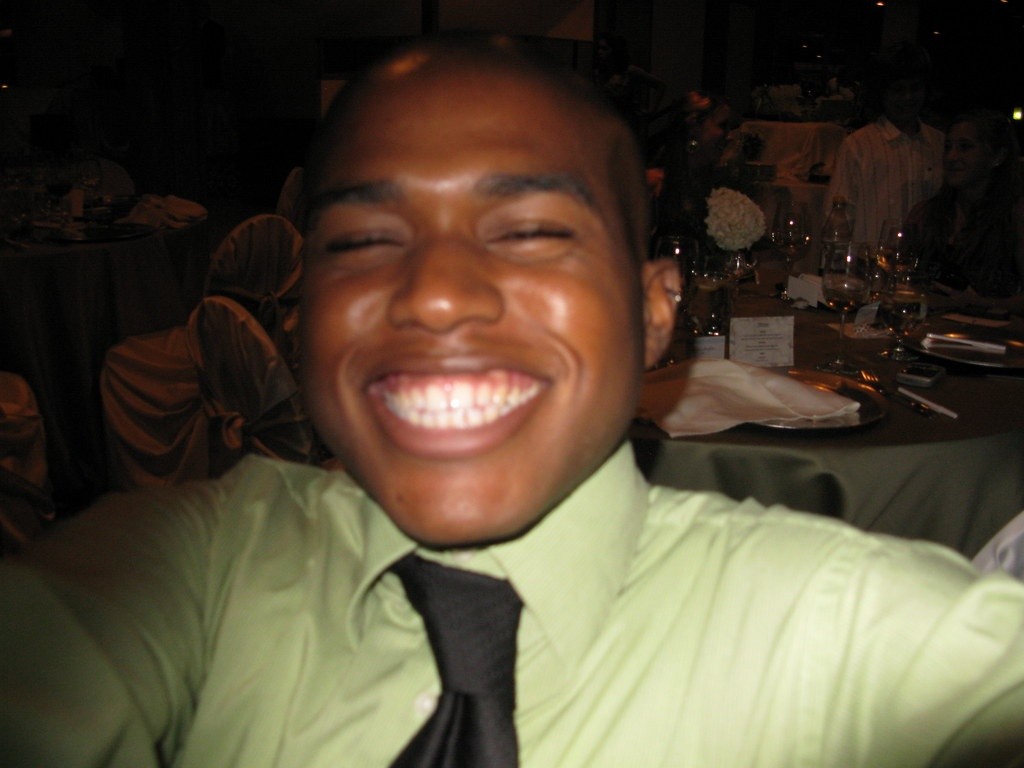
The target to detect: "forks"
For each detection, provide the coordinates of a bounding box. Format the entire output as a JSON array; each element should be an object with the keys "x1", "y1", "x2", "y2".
[{"x1": 858, "y1": 370, "x2": 944, "y2": 426}]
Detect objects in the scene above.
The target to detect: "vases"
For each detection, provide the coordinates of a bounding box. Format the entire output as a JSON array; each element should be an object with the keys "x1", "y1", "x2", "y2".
[{"x1": 725, "y1": 249, "x2": 755, "y2": 304}]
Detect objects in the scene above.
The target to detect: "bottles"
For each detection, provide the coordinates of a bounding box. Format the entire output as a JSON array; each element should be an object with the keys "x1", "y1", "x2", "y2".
[{"x1": 817, "y1": 196, "x2": 852, "y2": 279}]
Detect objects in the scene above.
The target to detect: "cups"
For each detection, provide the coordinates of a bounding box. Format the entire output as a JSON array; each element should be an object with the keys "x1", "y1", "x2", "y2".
[
  {"x1": 875, "y1": 218, "x2": 920, "y2": 282},
  {"x1": 652, "y1": 236, "x2": 698, "y2": 276},
  {"x1": 683, "y1": 271, "x2": 733, "y2": 334}
]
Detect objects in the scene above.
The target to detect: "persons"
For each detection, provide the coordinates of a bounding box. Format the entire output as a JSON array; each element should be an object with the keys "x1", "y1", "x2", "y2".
[
  {"x1": 187, "y1": 3, "x2": 229, "y2": 174},
  {"x1": 896, "y1": 108, "x2": 1024, "y2": 315},
  {"x1": 732, "y1": 131, "x2": 776, "y2": 197},
  {"x1": 0, "y1": 30, "x2": 1024, "y2": 768},
  {"x1": 816, "y1": 40, "x2": 947, "y2": 276},
  {"x1": 590, "y1": 35, "x2": 666, "y2": 153},
  {"x1": 639, "y1": 88, "x2": 776, "y2": 287}
]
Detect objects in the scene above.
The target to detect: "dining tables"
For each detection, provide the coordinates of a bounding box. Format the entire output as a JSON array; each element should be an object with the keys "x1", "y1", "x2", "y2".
[
  {"x1": 0, "y1": 197, "x2": 207, "y2": 519},
  {"x1": 629, "y1": 261, "x2": 1024, "y2": 561}
]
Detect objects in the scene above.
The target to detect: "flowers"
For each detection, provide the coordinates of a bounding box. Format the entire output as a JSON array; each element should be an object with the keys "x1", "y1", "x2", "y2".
[{"x1": 704, "y1": 186, "x2": 767, "y2": 253}]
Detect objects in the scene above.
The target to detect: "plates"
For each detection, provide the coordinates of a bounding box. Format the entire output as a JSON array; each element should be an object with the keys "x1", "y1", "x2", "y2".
[
  {"x1": 744, "y1": 363, "x2": 889, "y2": 432},
  {"x1": 903, "y1": 321, "x2": 1024, "y2": 368}
]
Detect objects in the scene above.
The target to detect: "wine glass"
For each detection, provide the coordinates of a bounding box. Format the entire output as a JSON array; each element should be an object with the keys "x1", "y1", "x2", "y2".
[
  {"x1": 875, "y1": 274, "x2": 930, "y2": 363},
  {"x1": 816, "y1": 241, "x2": 872, "y2": 377},
  {"x1": 772, "y1": 200, "x2": 812, "y2": 302}
]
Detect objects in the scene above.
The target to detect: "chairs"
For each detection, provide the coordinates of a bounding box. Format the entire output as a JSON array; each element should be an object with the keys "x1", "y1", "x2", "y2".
[{"x1": 0, "y1": 165, "x2": 324, "y2": 559}]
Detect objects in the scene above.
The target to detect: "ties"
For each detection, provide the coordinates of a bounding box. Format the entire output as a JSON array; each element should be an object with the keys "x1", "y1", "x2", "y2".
[{"x1": 383, "y1": 553, "x2": 525, "y2": 768}]
